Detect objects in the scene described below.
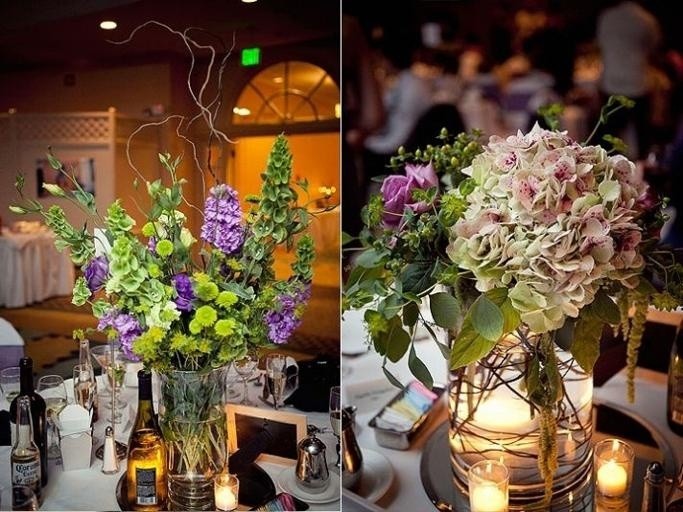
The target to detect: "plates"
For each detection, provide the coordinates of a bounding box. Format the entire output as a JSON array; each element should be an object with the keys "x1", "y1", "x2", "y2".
[
  {"x1": 277, "y1": 464, "x2": 340, "y2": 504},
  {"x1": 350, "y1": 448, "x2": 396, "y2": 502},
  {"x1": 418, "y1": 397, "x2": 675, "y2": 512},
  {"x1": 114, "y1": 453, "x2": 276, "y2": 510}
]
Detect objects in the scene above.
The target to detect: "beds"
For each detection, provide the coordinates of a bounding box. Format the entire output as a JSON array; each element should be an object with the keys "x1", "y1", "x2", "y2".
[{"x1": 0, "y1": 222, "x2": 73, "y2": 308}]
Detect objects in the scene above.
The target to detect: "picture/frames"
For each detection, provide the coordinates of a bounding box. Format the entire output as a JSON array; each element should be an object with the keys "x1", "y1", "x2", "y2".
[{"x1": 224, "y1": 403, "x2": 308, "y2": 467}]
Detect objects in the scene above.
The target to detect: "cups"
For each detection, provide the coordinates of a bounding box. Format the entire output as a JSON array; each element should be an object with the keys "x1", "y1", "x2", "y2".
[
  {"x1": 468, "y1": 460, "x2": 511, "y2": 512},
  {"x1": 212, "y1": 472, "x2": 240, "y2": 510},
  {"x1": 0, "y1": 484, "x2": 39, "y2": 512},
  {"x1": 0, "y1": 368, "x2": 24, "y2": 405},
  {"x1": 591, "y1": 437, "x2": 637, "y2": 507}
]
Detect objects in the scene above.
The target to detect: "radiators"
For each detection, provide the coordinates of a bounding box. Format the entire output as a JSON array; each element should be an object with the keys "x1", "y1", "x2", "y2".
[{"x1": 341, "y1": 87, "x2": 683, "y2": 504}]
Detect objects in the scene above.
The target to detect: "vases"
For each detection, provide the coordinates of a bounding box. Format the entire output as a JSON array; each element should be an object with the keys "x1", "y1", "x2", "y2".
[
  {"x1": 441, "y1": 324, "x2": 597, "y2": 512},
  {"x1": 156, "y1": 362, "x2": 227, "y2": 508}
]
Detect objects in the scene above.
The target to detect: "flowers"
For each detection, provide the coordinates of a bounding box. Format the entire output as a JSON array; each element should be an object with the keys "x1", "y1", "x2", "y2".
[{"x1": 7, "y1": 19, "x2": 342, "y2": 486}]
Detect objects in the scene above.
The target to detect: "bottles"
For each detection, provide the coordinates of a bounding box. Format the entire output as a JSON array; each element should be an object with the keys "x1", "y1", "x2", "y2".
[
  {"x1": 120, "y1": 367, "x2": 164, "y2": 509},
  {"x1": 641, "y1": 461, "x2": 666, "y2": 512},
  {"x1": 666, "y1": 320, "x2": 683, "y2": 435},
  {"x1": 77, "y1": 338, "x2": 98, "y2": 424},
  {"x1": 343, "y1": 405, "x2": 365, "y2": 488},
  {"x1": 8, "y1": 355, "x2": 48, "y2": 511},
  {"x1": 100, "y1": 426, "x2": 122, "y2": 476},
  {"x1": 293, "y1": 437, "x2": 331, "y2": 493}
]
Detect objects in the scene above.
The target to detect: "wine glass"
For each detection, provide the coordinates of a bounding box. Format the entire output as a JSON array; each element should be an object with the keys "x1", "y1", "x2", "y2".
[
  {"x1": 327, "y1": 385, "x2": 341, "y2": 468},
  {"x1": 90, "y1": 343, "x2": 129, "y2": 424},
  {"x1": 37, "y1": 374, "x2": 67, "y2": 458},
  {"x1": 70, "y1": 363, "x2": 99, "y2": 447},
  {"x1": 226, "y1": 345, "x2": 288, "y2": 413}
]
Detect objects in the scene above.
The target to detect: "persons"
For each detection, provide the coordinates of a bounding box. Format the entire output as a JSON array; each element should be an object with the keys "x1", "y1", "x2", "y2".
[{"x1": 341, "y1": 2, "x2": 682, "y2": 276}]
